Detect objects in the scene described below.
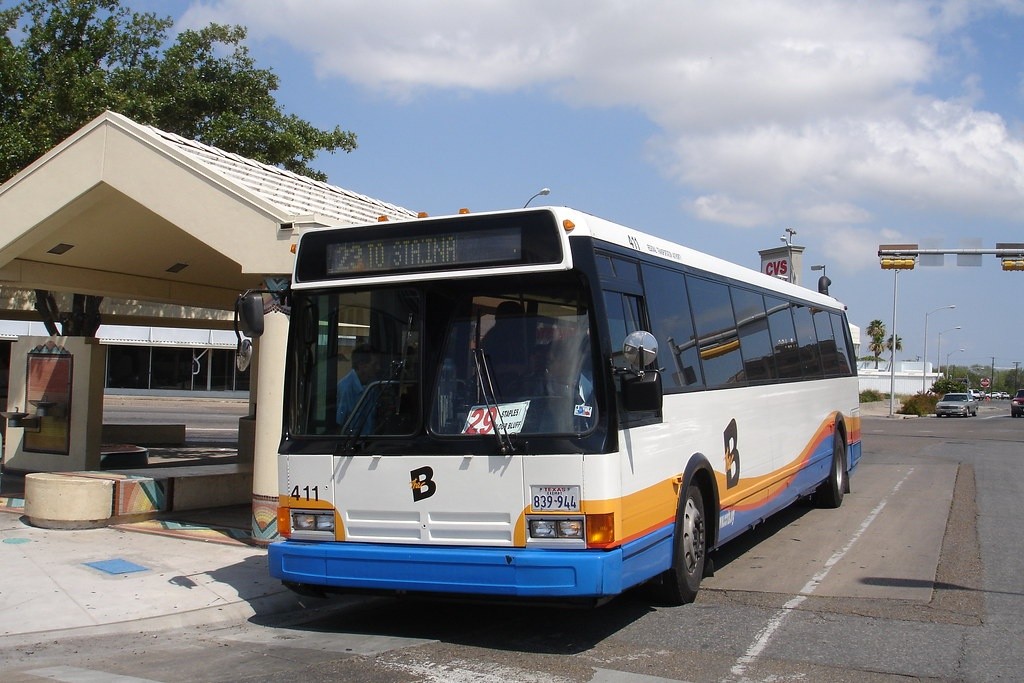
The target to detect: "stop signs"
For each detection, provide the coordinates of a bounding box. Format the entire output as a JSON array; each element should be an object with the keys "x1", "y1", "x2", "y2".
[{"x1": 981, "y1": 379, "x2": 989, "y2": 387}]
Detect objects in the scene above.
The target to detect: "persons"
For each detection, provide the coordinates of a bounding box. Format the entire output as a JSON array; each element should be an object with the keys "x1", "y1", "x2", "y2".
[
  {"x1": 480, "y1": 301, "x2": 594, "y2": 433},
  {"x1": 336, "y1": 344, "x2": 381, "y2": 435}
]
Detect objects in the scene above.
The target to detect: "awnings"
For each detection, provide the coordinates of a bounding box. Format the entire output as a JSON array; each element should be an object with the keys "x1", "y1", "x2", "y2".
[{"x1": 0, "y1": 320, "x2": 356, "y2": 351}]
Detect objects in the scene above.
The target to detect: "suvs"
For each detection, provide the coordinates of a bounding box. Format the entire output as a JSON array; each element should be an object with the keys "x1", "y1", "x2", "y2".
[{"x1": 969, "y1": 389, "x2": 980, "y2": 400}]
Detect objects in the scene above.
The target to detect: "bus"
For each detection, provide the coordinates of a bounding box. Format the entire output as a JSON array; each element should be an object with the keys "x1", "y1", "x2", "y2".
[{"x1": 231, "y1": 205, "x2": 864, "y2": 609}]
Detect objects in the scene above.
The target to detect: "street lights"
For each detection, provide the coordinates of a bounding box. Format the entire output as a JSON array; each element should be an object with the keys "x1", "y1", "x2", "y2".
[
  {"x1": 922, "y1": 305, "x2": 955, "y2": 394},
  {"x1": 1012, "y1": 361, "x2": 1022, "y2": 392},
  {"x1": 781, "y1": 237, "x2": 792, "y2": 282},
  {"x1": 810, "y1": 265, "x2": 825, "y2": 276},
  {"x1": 937, "y1": 326, "x2": 961, "y2": 383},
  {"x1": 890, "y1": 260, "x2": 920, "y2": 416},
  {"x1": 947, "y1": 349, "x2": 964, "y2": 379}
]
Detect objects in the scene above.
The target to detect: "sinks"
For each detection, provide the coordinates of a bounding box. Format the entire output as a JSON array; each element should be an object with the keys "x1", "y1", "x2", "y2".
[
  {"x1": 28, "y1": 400, "x2": 57, "y2": 409},
  {"x1": 0, "y1": 412, "x2": 29, "y2": 420}
]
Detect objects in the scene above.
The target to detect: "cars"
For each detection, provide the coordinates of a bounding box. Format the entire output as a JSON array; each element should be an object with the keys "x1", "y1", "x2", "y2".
[
  {"x1": 985, "y1": 392, "x2": 1002, "y2": 399},
  {"x1": 980, "y1": 391, "x2": 986, "y2": 400},
  {"x1": 935, "y1": 391, "x2": 978, "y2": 417},
  {"x1": 1011, "y1": 389, "x2": 1024, "y2": 418}
]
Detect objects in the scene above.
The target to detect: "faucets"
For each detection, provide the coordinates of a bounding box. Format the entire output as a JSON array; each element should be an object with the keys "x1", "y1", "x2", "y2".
[
  {"x1": 43, "y1": 393, "x2": 48, "y2": 402},
  {"x1": 15, "y1": 407, "x2": 18, "y2": 413}
]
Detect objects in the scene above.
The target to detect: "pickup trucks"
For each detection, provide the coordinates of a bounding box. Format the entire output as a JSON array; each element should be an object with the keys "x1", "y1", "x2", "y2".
[{"x1": 1000, "y1": 392, "x2": 1010, "y2": 399}]
